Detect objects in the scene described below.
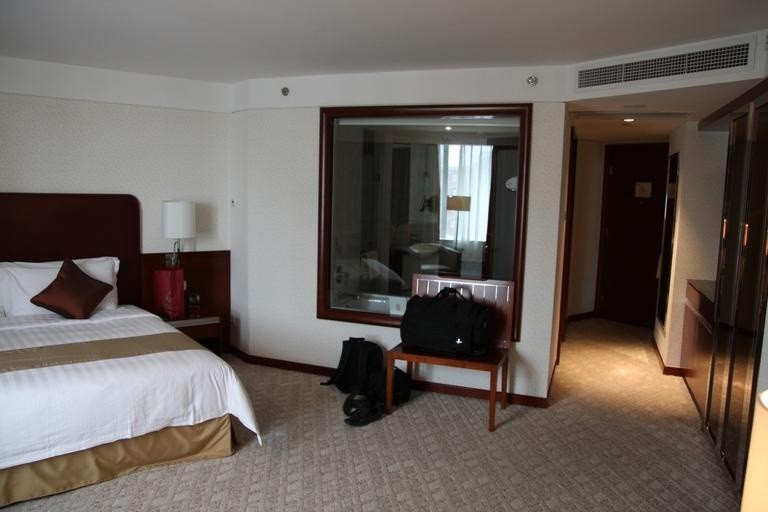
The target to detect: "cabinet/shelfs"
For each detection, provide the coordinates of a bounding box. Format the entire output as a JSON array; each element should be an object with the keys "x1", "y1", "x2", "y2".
[
  {"x1": 679, "y1": 279, "x2": 716, "y2": 415},
  {"x1": 391, "y1": 246, "x2": 409, "y2": 294},
  {"x1": 705, "y1": 104, "x2": 768, "y2": 488}
]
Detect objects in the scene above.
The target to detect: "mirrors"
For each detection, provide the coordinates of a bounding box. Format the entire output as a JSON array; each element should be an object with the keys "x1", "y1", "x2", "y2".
[{"x1": 317, "y1": 104, "x2": 531, "y2": 342}]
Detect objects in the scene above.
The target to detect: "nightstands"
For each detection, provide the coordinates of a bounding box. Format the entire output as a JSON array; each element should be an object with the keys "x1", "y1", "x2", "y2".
[{"x1": 162, "y1": 305, "x2": 221, "y2": 357}]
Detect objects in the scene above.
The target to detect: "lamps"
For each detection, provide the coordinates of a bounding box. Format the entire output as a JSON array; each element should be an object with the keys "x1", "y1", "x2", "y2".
[
  {"x1": 161, "y1": 201, "x2": 196, "y2": 265},
  {"x1": 447, "y1": 195, "x2": 472, "y2": 250}
]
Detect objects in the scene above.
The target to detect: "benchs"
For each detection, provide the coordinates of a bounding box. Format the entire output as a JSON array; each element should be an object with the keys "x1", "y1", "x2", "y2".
[{"x1": 385, "y1": 273, "x2": 515, "y2": 430}]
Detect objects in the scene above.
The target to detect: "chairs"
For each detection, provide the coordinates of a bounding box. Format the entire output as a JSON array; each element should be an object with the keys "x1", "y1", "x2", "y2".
[{"x1": 409, "y1": 243, "x2": 462, "y2": 289}]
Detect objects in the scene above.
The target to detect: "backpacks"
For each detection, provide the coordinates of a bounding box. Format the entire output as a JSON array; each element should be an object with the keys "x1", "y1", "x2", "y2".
[{"x1": 320, "y1": 337, "x2": 386, "y2": 396}]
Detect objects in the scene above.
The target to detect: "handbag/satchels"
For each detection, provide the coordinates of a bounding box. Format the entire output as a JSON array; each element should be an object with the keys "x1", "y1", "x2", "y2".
[{"x1": 399, "y1": 287, "x2": 496, "y2": 361}]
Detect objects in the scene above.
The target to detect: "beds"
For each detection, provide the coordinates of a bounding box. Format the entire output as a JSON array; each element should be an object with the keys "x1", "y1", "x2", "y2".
[{"x1": 0, "y1": 195, "x2": 260, "y2": 508}]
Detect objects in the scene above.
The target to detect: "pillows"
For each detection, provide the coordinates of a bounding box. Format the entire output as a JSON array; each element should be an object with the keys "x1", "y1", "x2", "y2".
[
  {"x1": 0, "y1": 255, "x2": 120, "y2": 317},
  {"x1": 32, "y1": 256, "x2": 113, "y2": 319}
]
